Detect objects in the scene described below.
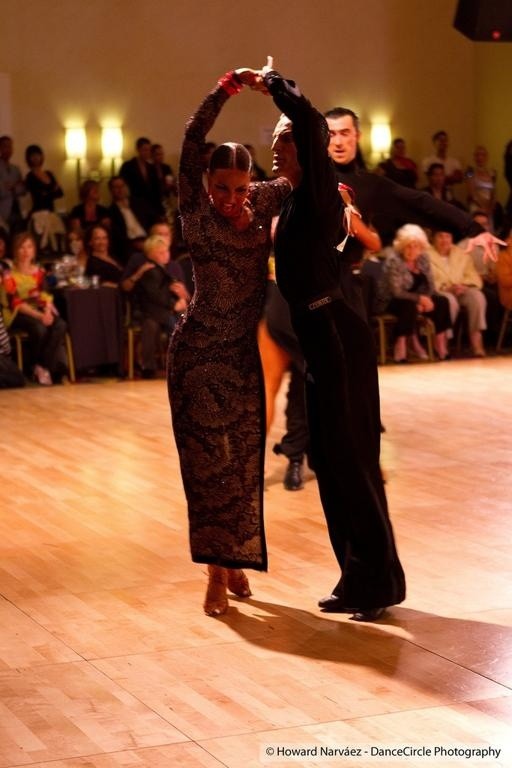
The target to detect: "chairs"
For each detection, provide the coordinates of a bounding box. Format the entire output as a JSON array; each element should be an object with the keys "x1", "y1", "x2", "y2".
[
  {"x1": 10, "y1": 331, "x2": 76, "y2": 384},
  {"x1": 360, "y1": 256, "x2": 434, "y2": 365},
  {"x1": 119, "y1": 297, "x2": 167, "y2": 379}
]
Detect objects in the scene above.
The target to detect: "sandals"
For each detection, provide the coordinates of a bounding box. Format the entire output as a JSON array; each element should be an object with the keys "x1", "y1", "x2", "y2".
[
  {"x1": 228, "y1": 568, "x2": 253, "y2": 600},
  {"x1": 203, "y1": 571, "x2": 229, "y2": 618}
]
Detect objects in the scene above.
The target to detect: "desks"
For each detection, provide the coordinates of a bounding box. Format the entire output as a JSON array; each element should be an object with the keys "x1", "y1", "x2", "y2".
[{"x1": 56, "y1": 278, "x2": 119, "y2": 373}]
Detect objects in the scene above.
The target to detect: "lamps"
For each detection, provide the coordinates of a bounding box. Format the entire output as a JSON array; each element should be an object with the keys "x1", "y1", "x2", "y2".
[
  {"x1": 370, "y1": 124, "x2": 392, "y2": 162},
  {"x1": 65, "y1": 120, "x2": 122, "y2": 189}
]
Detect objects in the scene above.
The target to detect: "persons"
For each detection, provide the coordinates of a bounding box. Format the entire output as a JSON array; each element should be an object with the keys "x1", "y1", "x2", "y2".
[{"x1": 0, "y1": 55, "x2": 512, "y2": 622}]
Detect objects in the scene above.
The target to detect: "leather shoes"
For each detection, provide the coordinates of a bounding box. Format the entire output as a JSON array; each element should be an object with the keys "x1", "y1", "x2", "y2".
[
  {"x1": 353, "y1": 606, "x2": 386, "y2": 623},
  {"x1": 284, "y1": 460, "x2": 302, "y2": 492},
  {"x1": 318, "y1": 594, "x2": 344, "y2": 612}
]
